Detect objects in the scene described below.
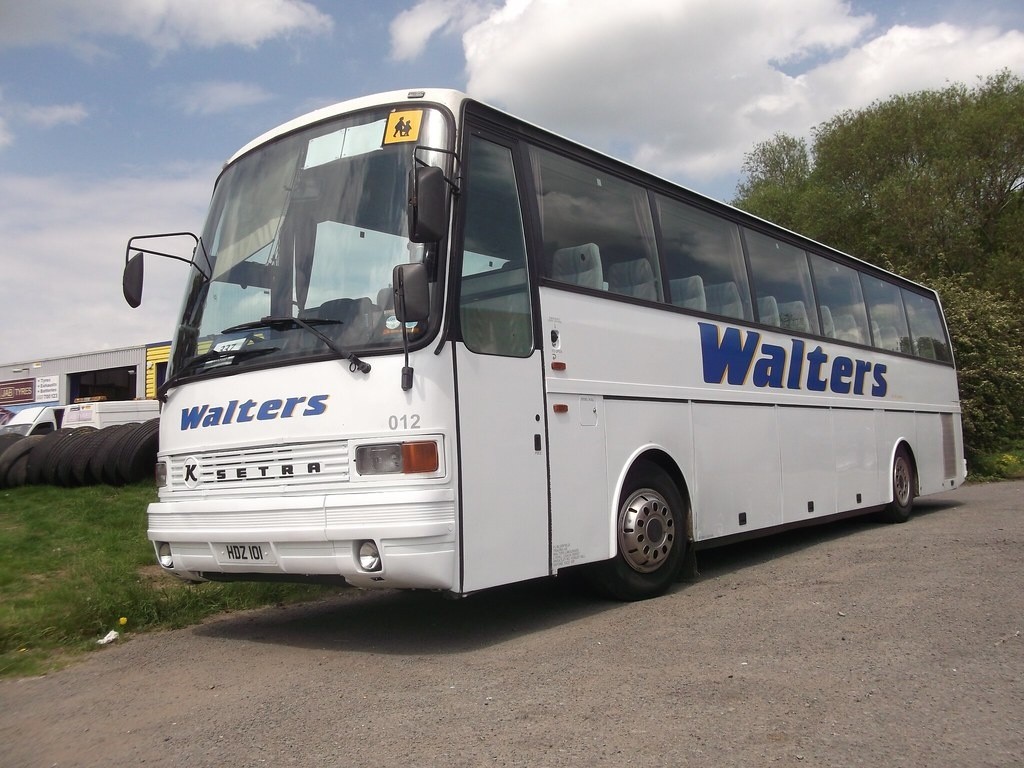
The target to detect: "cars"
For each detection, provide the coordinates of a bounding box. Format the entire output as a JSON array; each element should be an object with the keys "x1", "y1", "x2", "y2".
[{"x1": 1, "y1": 408, "x2": 16, "y2": 426}]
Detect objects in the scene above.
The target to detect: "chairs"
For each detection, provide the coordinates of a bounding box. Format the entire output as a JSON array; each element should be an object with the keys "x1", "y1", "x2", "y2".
[
  {"x1": 900, "y1": 331, "x2": 920, "y2": 356},
  {"x1": 668, "y1": 274, "x2": 707, "y2": 312},
  {"x1": 312, "y1": 297, "x2": 362, "y2": 348},
  {"x1": 820, "y1": 306, "x2": 836, "y2": 338},
  {"x1": 551, "y1": 243, "x2": 603, "y2": 290},
  {"x1": 757, "y1": 295, "x2": 780, "y2": 327},
  {"x1": 880, "y1": 326, "x2": 900, "y2": 352},
  {"x1": 607, "y1": 258, "x2": 658, "y2": 302},
  {"x1": 704, "y1": 282, "x2": 744, "y2": 319},
  {"x1": 777, "y1": 300, "x2": 810, "y2": 333},
  {"x1": 871, "y1": 321, "x2": 883, "y2": 349},
  {"x1": 918, "y1": 336, "x2": 937, "y2": 360},
  {"x1": 376, "y1": 287, "x2": 396, "y2": 311},
  {"x1": 832, "y1": 315, "x2": 860, "y2": 342}
]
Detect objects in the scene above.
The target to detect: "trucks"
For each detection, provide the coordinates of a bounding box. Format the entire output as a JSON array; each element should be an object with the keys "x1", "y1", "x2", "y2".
[
  {"x1": 0, "y1": 404, "x2": 67, "y2": 437},
  {"x1": 62, "y1": 400, "x2": 163, "y2": 430}
]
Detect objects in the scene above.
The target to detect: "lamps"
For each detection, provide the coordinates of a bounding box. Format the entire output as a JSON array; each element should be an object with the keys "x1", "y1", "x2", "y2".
[{"x1": 12, "y1": 367, "x2": 30, "y2": 373}]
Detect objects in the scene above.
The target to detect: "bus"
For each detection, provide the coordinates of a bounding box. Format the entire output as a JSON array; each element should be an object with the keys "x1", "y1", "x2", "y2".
[{"x1": 120, "y1": 85, "x2": 971, "y2": 598}]
